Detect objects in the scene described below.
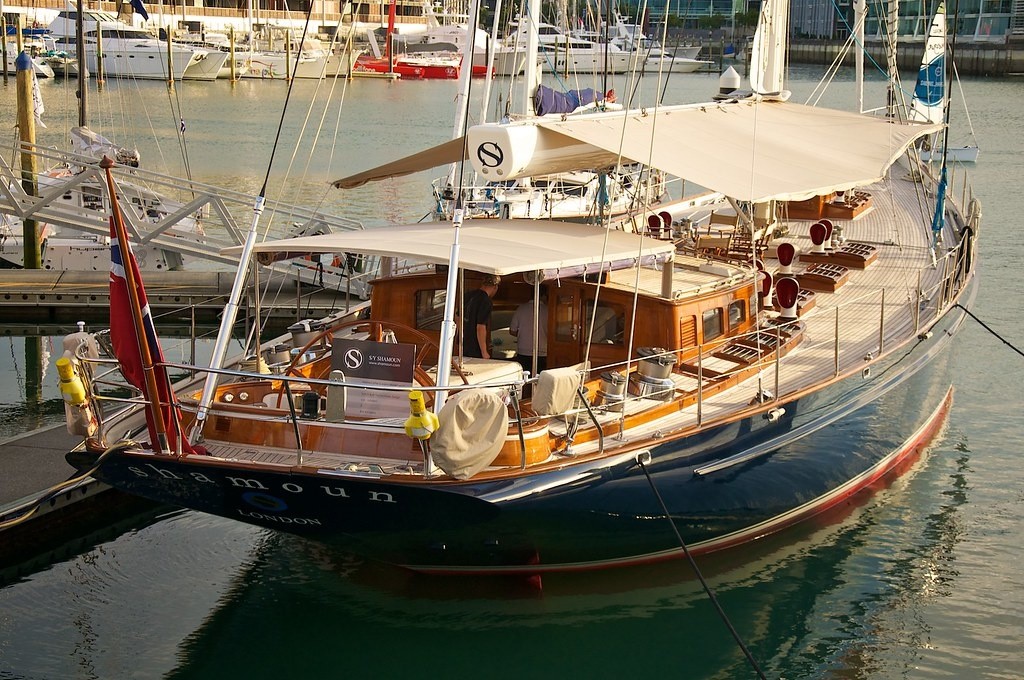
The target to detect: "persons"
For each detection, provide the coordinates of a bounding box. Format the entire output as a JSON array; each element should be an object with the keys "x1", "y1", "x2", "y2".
[
  {"x1": 454, "y1": 275, "x2": 501, "y2": 359},
  {"x1": 510, "y1": 284, "x2": 549, "y2": 399}
]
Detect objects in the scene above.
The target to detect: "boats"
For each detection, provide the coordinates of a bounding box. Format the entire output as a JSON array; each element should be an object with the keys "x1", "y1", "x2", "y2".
[{"x1": 1, "y1": 0, "x2": 717, "y2": 81}]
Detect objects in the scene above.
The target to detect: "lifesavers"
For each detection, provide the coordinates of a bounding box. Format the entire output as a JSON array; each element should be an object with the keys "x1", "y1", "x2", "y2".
[{"x1": 304, "y1": 254, "x2": 341, "y2": 274}]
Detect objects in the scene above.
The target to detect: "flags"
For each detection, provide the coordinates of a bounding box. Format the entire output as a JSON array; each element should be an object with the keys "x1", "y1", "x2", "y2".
[
  {"x1": 128, "y1": 0, "x2": 149, "y2": 21},
  {"x1": 31, "y1": 65, "x2": 46, "y2": 128},
  {"x1": 107, "y1": 206, "x2": 208, "y2": 455}
]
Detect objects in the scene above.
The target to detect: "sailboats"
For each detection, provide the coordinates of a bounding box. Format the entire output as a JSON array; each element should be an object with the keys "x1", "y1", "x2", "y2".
[
  {"x1": 0, "y1": 0, "x2": 207, "y2": 271},
  {"x1": 904, "y1": 0, "x2": 980, "y2": 163},
  {"x1": 55, "y1": 0, "x2": 984, "y2": 582}
]
